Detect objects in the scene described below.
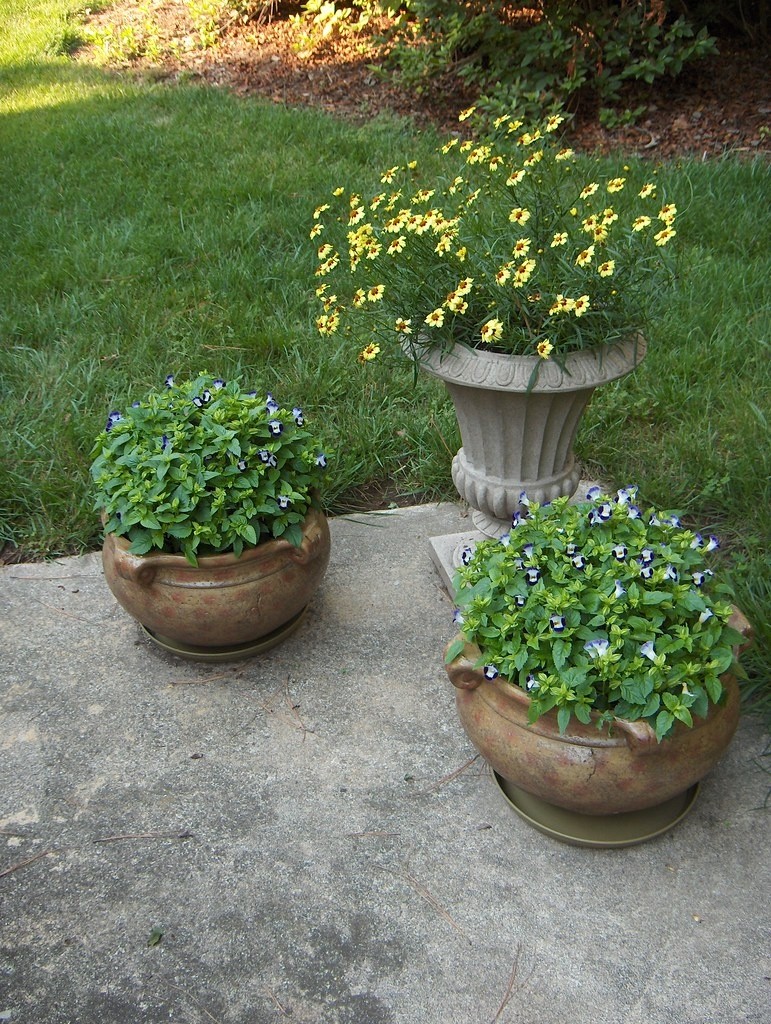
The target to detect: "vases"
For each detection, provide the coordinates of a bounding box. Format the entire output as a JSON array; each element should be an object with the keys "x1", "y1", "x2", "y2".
[
  {"x1": 442, "y1": 603, "x2": 752, "y2": 847},
  {"x1": 395, "y1": 324, "x2": 649, "y2": 574},
  {"x1": 104, "y1": 507, "x2": 336, "y2": 662}
]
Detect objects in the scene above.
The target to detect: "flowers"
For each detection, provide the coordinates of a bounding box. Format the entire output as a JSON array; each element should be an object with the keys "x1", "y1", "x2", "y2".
[
  {"x1": 450, "y1": 477, "x2": 723, "y2": 739},
  {"x1": 93, "y1": 371, "x2": 329, "y2": 556},
  {"x1": 312, "y1": 102, "x2": 681, "y2": 378}
]
